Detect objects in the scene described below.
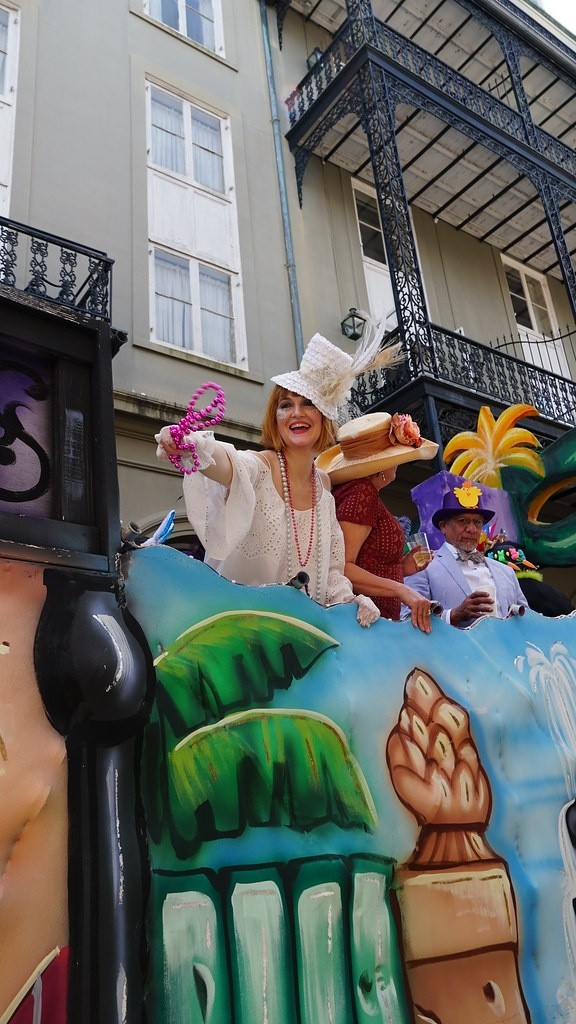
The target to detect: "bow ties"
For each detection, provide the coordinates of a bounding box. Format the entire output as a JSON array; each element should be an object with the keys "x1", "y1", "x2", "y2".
[{"x1": 457, "y1": 549, "x2": 485, "y2": 563}]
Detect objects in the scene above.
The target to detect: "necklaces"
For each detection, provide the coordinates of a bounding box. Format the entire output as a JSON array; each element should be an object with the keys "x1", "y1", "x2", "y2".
[
  {"x1": 163, "y1": 380, "x2": 225, "y2": 474},
  {"x1": 276, "y1": 448, "x2": 322, "y2": 605}
]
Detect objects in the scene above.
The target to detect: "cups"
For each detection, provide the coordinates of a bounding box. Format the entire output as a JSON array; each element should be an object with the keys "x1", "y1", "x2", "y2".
[
  {"x1": 405, "y1": 531, "x2": 432, "y2": 566},
  {"x1": 473, "y1": 586, "x2": 497, "y2": 616}
]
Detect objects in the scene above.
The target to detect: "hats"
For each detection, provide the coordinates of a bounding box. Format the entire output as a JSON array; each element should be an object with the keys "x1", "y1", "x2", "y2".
[
  {"x1": 431, "y1": 489, "x2": 496, "y2": 530},
  {"x1": 314, "y1": 411, "x2": 440, "y2": 485},
  {"x1": 270, "y1": 333, "x2": 354, "y2": 421}
]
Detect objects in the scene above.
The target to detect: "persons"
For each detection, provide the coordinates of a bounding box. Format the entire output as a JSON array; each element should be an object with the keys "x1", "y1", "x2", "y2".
[
  {"x1": 318, "y1": 413, "x2": 432, "y2": 634},
  {"x1": 399, "y1": 490, "x2": 529, "y2": 628},
  {"x1": 157, "y1": 335, "x2": 381, "y2": 627}
]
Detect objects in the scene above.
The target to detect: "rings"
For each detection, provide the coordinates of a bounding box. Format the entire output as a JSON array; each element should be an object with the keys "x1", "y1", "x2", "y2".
[{"x1": 426, "y1": 608, "x2": 431, "y2": 617}]
